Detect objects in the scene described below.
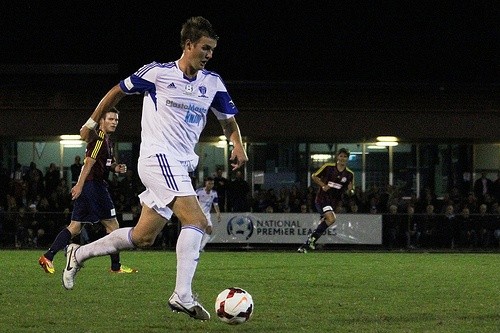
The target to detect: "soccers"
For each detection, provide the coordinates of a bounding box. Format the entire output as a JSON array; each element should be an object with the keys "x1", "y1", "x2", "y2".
[{"x1": 214, "y1": 287, "x2": 255, "y2": 324}]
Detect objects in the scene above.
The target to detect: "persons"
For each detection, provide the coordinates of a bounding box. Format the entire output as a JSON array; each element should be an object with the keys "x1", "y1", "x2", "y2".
[
  {"x1": 192, "y1": 176, "x2": 223, "y2": 254},
  {"x1": 0, "y1": 156, "x2": 500, "y2": 249},
  {"x1": 37, "y1": 107, "x2": 139, "y2": 274},
  {"x1": 298, "y1": 148, "x2": 355, "y2": 253},
  {"x1": 61, "y1": 16, "x2": 249, "y2": 322}
]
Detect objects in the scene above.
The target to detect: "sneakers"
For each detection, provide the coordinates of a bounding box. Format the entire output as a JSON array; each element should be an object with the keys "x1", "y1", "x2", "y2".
[
  {"x1": 109, "y1": 264, "x2": 139, "y2": 274},
  {"x1": 39, "y1": 255, "x2": 56, "y2": 274},
  {"x1": 307, "y1": 238, "x2": 316, "y2": 250},
  {"x1": 297, "y1": 248, "x2": 307, "y2": 254},
  {"x1": 62, "y1": 243, "x2": 85, "y2": 290},
  {"x1": 167, "y1": 290, "x2": 211, "y2": 324}
]
